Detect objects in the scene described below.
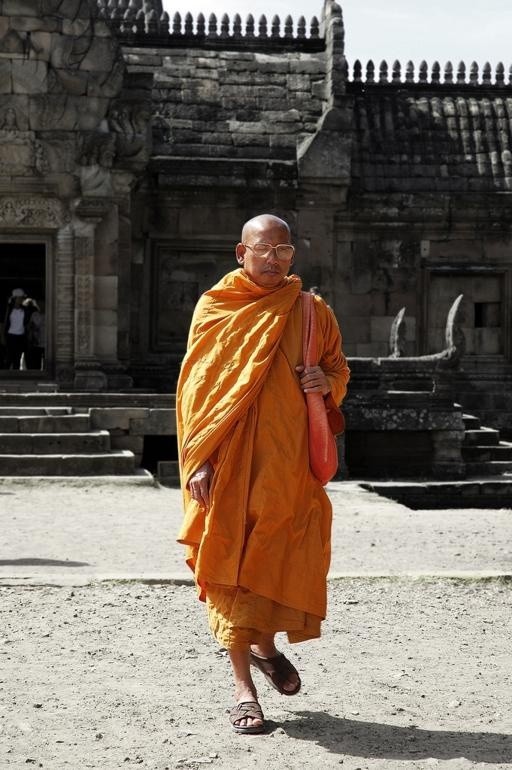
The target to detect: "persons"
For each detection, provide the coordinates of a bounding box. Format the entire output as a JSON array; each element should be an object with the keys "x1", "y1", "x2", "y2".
[
  {"x1": 176, "y1": 212, "x2": 351, "y2": 737},
  {"x1": 1, "y1": 285, "x2": 45, "y2": 370}
]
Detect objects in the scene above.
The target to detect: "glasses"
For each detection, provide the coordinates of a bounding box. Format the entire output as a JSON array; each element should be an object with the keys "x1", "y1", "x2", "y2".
[{"x1": 240, "y1": 242, "x2": 295, "y2": 261}]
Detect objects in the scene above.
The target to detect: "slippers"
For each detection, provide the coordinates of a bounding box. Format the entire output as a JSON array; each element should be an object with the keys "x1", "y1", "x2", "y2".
[
  {"x1": 250, "y1": 643, "x2": 301, "y2": 695},
  {"x1": 229, "y1": 702, "x2": 266, "y2": 733}
]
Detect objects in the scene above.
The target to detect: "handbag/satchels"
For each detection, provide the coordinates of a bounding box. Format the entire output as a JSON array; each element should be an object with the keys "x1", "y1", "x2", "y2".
[{"x1": 305, "y1": 291, "x2": 339, "y2": 486}]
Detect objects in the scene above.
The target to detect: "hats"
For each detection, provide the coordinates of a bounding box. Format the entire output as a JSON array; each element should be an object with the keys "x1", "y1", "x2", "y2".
[{"x1": 8, "y1": 288, "x2": 28, "y2": 304}]
